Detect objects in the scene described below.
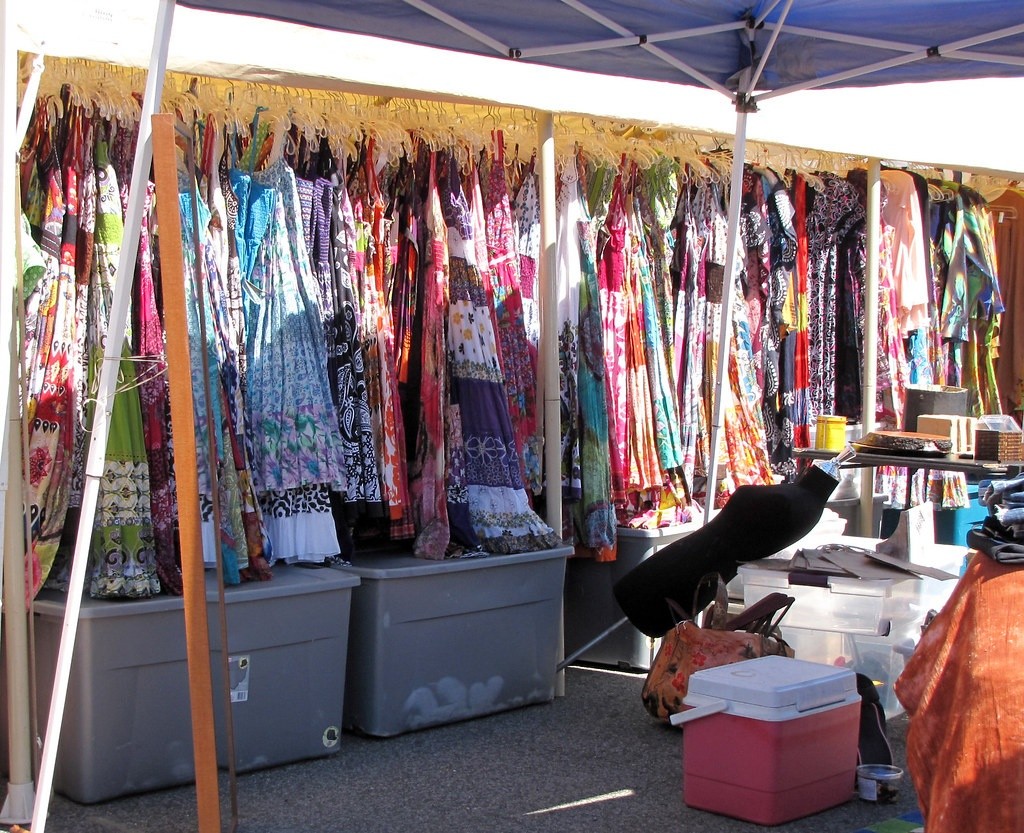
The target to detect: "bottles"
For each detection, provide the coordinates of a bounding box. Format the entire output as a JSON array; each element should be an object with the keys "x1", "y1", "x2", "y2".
[{"x1": 811, "y1": 446, "x2": 856, "y2": 482}]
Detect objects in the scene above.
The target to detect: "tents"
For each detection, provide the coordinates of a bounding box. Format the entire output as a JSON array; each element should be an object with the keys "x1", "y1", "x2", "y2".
[{"x1": 0, "y1": 0, "x2": 1024, "y2": 832}]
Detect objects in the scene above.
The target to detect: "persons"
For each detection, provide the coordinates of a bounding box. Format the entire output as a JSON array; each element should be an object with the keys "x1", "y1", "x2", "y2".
[{"x1": 613, "y1": 458, "x2": 843, "y2": 639}]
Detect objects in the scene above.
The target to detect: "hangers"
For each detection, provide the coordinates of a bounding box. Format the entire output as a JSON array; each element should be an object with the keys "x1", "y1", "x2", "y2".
[
  {"x1": 16, "y1": 51, "x2": 1024, "y2": 219},
  {"x1": 665, "y1": 592, "x2": 794, "y2": 639}
]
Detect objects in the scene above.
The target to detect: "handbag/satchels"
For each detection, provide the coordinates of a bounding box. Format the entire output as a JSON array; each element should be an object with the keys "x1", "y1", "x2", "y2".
[{"x1": 641, "y1": 619, "x2": 795, "y2": 723}]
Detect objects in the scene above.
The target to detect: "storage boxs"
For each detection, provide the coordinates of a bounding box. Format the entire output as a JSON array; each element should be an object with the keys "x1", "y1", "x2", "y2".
[
  {"x1": 330, "y1": 543, "x2": 575, "y2": 737},
  {"x1": 0, "y1": 563, "x2": 360, "y2": 803},
  {"x1": 700, "y1": 535, "x2": 967, "y2": 722},
  {"x1": 809, "y1": 493, "x2": 889, "y2": 536},
  {"x1": 564, "y1": 524, "x2": 701, "y2": 671},
  {"x1": 808, "y1": 421, "x2": 888, "y2": 499},
  {"x1": 670, "y1": 655, "x2": 862, "y2": 827},
  {"x1": 880, "y1": 483, "x2": 990, "y2": 547}
]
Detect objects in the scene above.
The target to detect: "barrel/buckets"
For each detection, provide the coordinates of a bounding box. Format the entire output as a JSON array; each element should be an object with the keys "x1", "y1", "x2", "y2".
[
  {"x1": 903, "y1": 384, "x2": 967, "y2": 434},
  {"x1": 816, "y1": 415, "x2": 846, "y2": 450}
]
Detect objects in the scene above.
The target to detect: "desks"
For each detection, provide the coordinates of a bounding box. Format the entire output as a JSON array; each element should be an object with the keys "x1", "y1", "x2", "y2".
[{"x1": 793, "y1": 447, "x2": 1024, "y2": 509}]
